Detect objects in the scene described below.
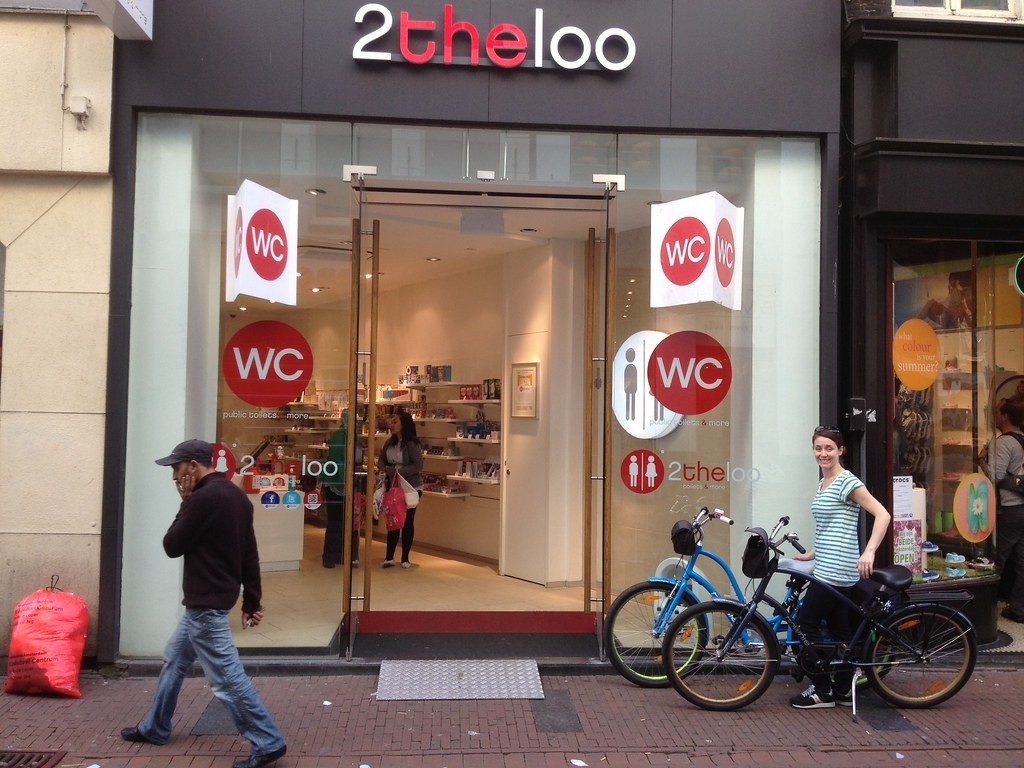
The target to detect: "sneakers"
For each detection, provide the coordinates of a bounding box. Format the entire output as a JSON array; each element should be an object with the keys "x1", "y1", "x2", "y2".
[
  {"x1": 945, "y1": 552, "x2": 965, "y2": 563},
  {"x1": 790, "y1": 685, "x2": 836, "y2": 709},
  {"x1": 831, "y1": 682, "x2": 852, "y2": 706},
  {"x1": 922, "y1": 568, "x2": 939, "y2": 581},
  {"x1": 946, "y1": 566, "x2": 966, "y2": 578},
  {"x1": 921, "y1": 541, "x2": 938, "y2": 553}
]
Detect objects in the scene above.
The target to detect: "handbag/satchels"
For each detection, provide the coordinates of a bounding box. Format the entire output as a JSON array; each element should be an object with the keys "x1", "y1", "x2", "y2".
[
  {"x1": 397, "y1": 469, "x2": 419, "y2": 508},
  {"x1": 383, "y1": 471, "x2": 407, "y2": 532}
]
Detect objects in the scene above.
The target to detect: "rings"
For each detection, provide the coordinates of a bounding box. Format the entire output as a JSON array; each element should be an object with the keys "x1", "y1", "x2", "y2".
[
  {"x1": 865, "y1": 567, "x2": 868, "y2": 568},
  {"x1": 181, "y1": 489, "x2": 185, "y2": 491}
]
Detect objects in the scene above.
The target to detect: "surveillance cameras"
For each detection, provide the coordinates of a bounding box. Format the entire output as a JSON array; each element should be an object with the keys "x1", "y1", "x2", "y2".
[{"x1": 229, "y1": 312, "x2": 236, "y2": 317}]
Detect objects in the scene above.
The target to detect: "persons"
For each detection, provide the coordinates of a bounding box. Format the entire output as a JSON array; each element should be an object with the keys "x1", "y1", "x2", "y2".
[
  {"x1": 376, "y1": 412, "x2": 423, "y2": 568},
  {"x1": 1010, "y1": 380, "x2": 1024, "y2": 400},
  {"x1": 322, "y1": 408, "x2": 349, "y2": 569},
  {"x1": 789, "y1": 425, "x2": 891, "y2": 708},
  {"x1": 120, "y1": 438, "x2": 287, "y2": 768},
  {"x1": 918, "y1": 270, "x2": 973, "y2": 329},
  {"x1": 978, "y1": 397, "x2": 1024, "y2": 624}
]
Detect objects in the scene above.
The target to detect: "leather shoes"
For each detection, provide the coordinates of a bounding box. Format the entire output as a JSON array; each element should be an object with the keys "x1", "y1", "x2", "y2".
[
  {"x1": 233, "y1": 745, "x2": 286, "y2": 768},
  {"x1": 120, "y1": 726, "x2": 147, "y2": 742}
]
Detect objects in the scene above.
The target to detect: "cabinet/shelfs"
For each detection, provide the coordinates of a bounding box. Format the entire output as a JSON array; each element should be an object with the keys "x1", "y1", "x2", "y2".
[
  {"x1": 923, "y1": 372, "x2": 975, "y2": 528},
  {"x1": 268, "y1": 380, "x2": 502, "y2": 500}
]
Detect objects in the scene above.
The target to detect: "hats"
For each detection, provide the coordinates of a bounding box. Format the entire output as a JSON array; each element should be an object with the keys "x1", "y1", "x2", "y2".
[{"x1": 155, "y1": 439, "x2": 213, "y2": 466}]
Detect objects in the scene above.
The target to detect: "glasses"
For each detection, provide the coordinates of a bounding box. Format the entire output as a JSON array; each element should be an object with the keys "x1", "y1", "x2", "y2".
[{"x1": 815, "y1": 426, "x2": 840, "y2": 434}]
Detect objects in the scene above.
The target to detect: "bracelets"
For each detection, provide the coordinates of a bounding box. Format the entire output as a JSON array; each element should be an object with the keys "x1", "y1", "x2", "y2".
[{"x1": 978, "y1": 457, "x2": 984, "y2": 460}]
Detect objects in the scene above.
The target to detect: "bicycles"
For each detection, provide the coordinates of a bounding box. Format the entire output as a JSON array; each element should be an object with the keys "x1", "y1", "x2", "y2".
[
  {"x1": 597, "y1": 502, "x2": 903, "y2": 697},
  {"x1": 659, "y1": 515, "x2": 978, "y2": 724}
]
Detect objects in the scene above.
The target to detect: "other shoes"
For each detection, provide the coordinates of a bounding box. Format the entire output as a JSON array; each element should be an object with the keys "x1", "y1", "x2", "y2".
[
  {"x1": 402, "y1": 561, "x2": 411, "y2": 568},
  {"x1": 352, "y1": 559, "x2": 360, "y2": 568},
  {"x1": 1002, "y1": 607, "x2": 1024, "y2": 623},
  {"x1": 943, "y1": 473, "x2": 960, "y2": 480},
  {"x1": 898, "y1": 383, "x2": 932, "y2": 493},
  {"x1": 382, "y1": 559, "x2": 396, "y2": 568}
]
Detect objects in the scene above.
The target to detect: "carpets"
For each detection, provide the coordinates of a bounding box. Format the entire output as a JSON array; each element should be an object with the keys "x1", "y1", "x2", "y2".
[{"x1": 342, "y1": 608, "x2": 600, "y2": 657}]
